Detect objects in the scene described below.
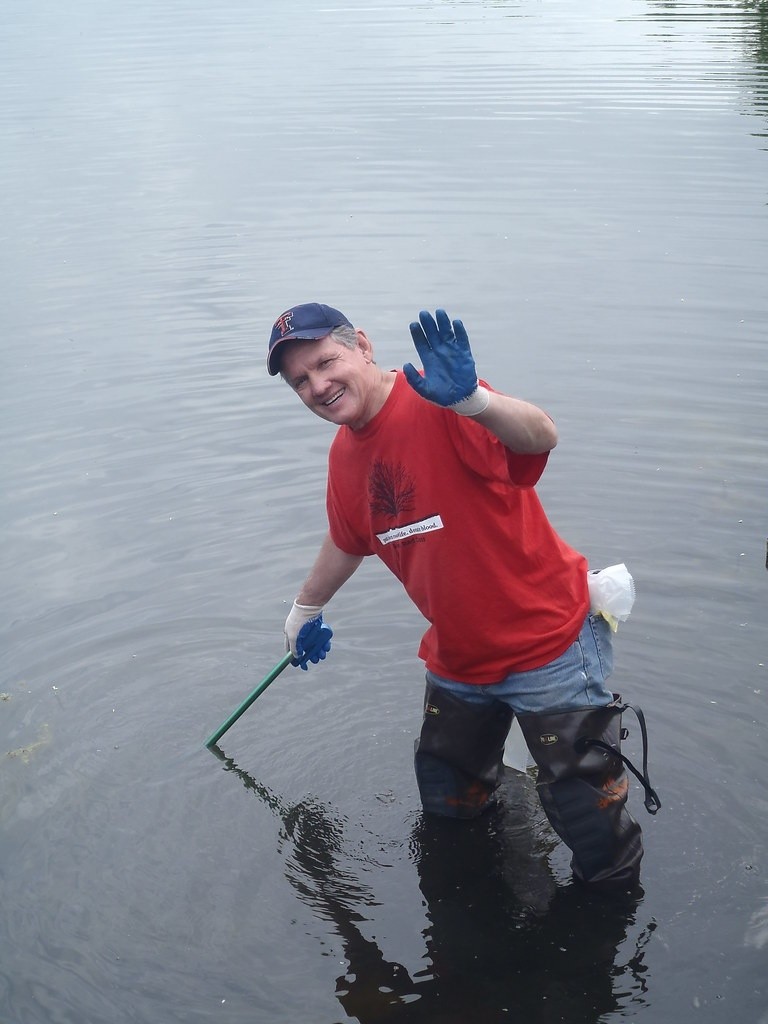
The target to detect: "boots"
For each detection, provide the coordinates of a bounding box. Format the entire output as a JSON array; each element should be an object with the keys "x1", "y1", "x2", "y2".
[
  {"x1": 414, "y1": 672, "x2": 515, "y2": 817},
  {"x1": 515, "y1": 693, "x2": 661, "y2": 892}
]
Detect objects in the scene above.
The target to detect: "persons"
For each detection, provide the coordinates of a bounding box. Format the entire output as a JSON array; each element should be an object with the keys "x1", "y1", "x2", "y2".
[{"x1": 267, "y1": 303, "x2": 662, "y2": 883}]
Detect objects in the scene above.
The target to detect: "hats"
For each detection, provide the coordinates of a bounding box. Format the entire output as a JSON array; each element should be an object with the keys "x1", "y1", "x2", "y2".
[{"x1": 267, "y1": 303, "x2": 354, "y2": 376}]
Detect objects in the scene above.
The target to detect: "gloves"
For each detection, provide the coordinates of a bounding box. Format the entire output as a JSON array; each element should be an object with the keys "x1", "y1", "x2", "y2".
[
  {"x1": 283, "y1": 597, "x2": 334, "y2": 671},
  {"x1": 403, "y1": 309, "x2": 490, "y2": 416}
]
